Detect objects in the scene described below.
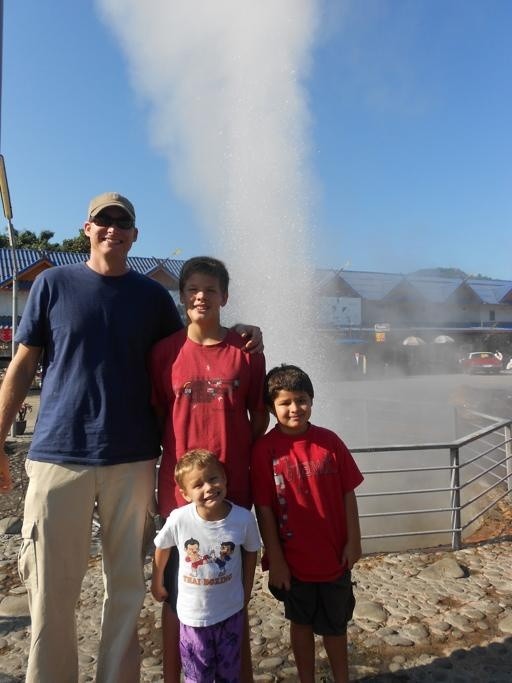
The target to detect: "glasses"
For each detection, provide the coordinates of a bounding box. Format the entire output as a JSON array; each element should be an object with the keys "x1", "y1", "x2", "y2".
[{"x1": 89, "y1": 214, "x2": 133, "y2": 229}]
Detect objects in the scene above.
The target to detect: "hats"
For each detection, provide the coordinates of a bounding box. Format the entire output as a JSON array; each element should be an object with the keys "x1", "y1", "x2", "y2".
[{"x1": 88, "y1": 192, "x2": 135, "y2": 221}]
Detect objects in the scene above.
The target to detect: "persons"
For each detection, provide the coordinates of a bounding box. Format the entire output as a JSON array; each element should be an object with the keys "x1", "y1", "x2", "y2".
[
  {"x1": 145, "y1": 257, "x2": 271, "y2": 683},
  {"x1": 248, "y1": 362, "x2": 366, "y2": 682},
  {"x1": 0, "y1": 192, "x2": 266, "y2": 683},
  {"x1": 495, "y1": 349, "x2": 503, "y2": 361},
  {"x1": 150, "y1": 448, "x2": 262, "y2": 683}
]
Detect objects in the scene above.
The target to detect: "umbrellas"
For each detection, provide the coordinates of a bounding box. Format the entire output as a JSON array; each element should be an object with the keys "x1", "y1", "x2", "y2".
[
  {"x1": 403, "y1": 336, "x2": 426, "y2": 346},
  {"x1": 434, "y1": 335, "x2": 455, "y2": 343}
]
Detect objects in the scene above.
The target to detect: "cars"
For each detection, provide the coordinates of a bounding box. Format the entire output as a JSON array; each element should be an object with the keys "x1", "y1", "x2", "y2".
[{"x1": 458, "y1": 352, "x2": 502, "y2": 376}]
